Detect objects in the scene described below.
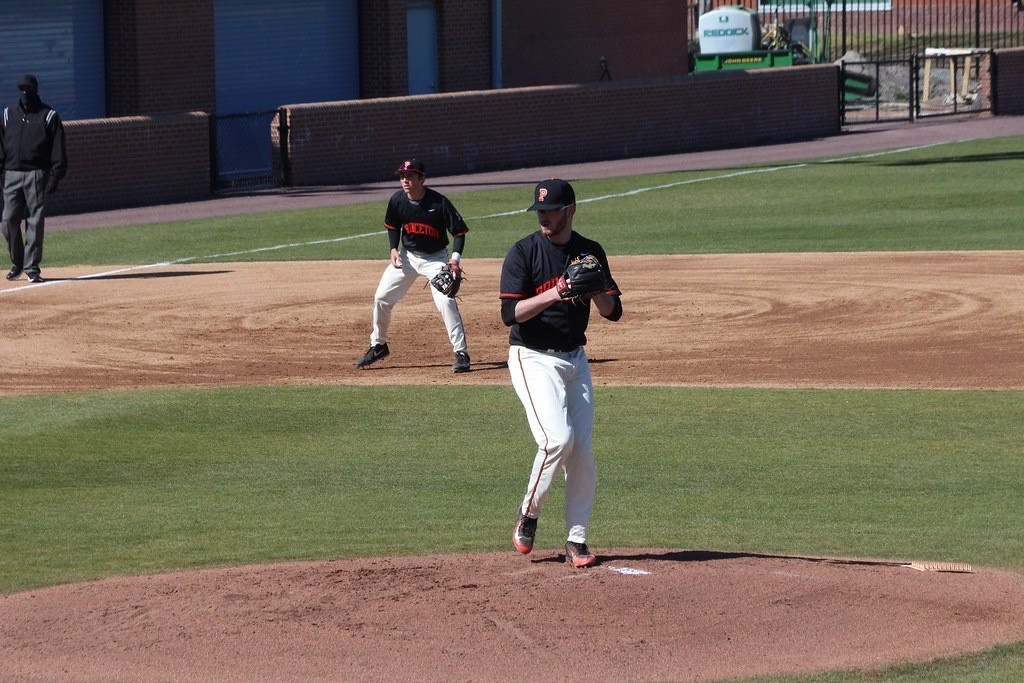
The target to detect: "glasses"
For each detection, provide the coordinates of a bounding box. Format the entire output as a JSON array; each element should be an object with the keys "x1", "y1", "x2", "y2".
[
  {"x1": 534, "y1": 203, "x2": 574, "y2": 215},
  {"x1": 19, "y1": 85, "x2": 34, "y2": 91}
]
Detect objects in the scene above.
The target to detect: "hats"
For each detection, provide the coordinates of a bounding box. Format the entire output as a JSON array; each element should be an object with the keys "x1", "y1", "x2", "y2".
[
  {"x1": 17, "y1": 75, "x2": 38, "y2": 89},
  {"x1": 526, "y1": 178, "x2": 576, "y2": 210},
  {"x1": 396, "y1": 159, "x2": 426, "y2": 178}
]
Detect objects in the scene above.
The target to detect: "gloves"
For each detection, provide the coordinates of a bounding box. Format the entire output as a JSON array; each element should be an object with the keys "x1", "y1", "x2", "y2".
[{"x1": 45, "y1": 174, "x2": 60, "y2": 195}]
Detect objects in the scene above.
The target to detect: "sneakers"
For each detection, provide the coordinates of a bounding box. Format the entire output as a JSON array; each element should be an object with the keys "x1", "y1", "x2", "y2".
[
  {"x1": 6, "y1": 264, "x2": 24, "y2": 279},
  {"x1": 512, "y1": 503, "x2": 537, "y2": 555},
  {"x1": 356, "y1": 341, "x2": 390, "y2": 368},
  {"x1": 25, "y1": 270, "x2": 43, "y2": 283},
  {"x1": 452, "y1": 351, "x2": 470, "y2": 370},
  {"x1": 565, "y1": 541, "x2": 596, "y2": 567}
]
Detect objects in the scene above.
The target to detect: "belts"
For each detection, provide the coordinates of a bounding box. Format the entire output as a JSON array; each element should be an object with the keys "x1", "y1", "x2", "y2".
[{"x1": 536, "y1": 346, "x2": 578, "y2": 354}]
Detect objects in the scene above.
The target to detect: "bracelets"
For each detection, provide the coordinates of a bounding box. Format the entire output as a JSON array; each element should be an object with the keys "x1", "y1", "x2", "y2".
[{"x1": 452, "y1": 252, "x2": 460, "y2": 263}]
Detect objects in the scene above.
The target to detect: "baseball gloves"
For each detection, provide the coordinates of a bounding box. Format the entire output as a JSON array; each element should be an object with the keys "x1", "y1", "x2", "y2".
[
  {"x1": 429, "y1": 262, "x2": 463, "y2": 298},
  {"x1": 556, "y1": 253, "x2": 623, "y2": 302}
]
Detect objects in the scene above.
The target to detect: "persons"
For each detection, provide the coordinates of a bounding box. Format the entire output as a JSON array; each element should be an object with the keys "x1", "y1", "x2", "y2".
[
  {"x1": 0, "y1": 75, "x2": 68, "y2": 282},
  {"x1": 499, "y1": 178, "x2": 623, "y2": 566},
  {"x1": 355, "y1": 159, "x2": 471, "y2": 373}
]
